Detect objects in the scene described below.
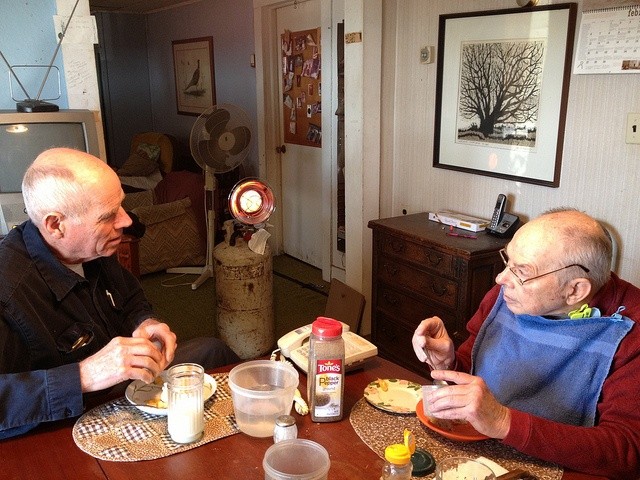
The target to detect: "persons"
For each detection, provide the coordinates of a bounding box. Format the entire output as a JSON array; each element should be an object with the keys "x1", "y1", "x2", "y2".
[
  {"x1": 1, "y1": 146, "x2": 178, "y2": 445},
  {"x1": 410, "y1": 207, "x2": 640, "y2": 474}
]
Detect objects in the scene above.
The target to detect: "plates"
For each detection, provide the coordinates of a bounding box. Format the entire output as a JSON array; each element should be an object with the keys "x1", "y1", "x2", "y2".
[
  {"x1": 364, "y1": 377, "x2": 424, "y2": 416},
  {"x1": 125, "y1": 367, "x2": 217, "y2": 416},
  {"x1": 416, "y1": 397, "x2": 493, "y2": 442}
]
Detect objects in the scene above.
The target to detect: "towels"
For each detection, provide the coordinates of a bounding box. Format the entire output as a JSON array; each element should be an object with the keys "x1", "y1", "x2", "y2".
[{"x1": 471, "y1": 286, "x2": 635, "y2": 427}]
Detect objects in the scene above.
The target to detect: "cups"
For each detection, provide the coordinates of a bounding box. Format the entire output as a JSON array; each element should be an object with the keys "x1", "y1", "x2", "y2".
[
  {"x1": 227, "y1": 360, "x2": 300, "y2": 439},
  {"x1": 261, "y1": 440, "x2": 332, "y2": 480},
  {"x1": 435, "y1": 456, "x2": 497, "y2": 480},
  {"x1": 166, "y1": 362, "x2": 205, "y2": 444}
]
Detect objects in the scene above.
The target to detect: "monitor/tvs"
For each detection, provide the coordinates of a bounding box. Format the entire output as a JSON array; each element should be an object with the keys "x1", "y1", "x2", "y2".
[{"x1": 0, "y1": 109, "x2": 108, "y2": 236}]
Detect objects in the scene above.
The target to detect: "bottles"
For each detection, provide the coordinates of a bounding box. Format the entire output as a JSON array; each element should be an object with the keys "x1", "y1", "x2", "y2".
[
  {"x1": 382, "y1": 427, "x2": 416, "y2": 479},
  {"x1": 307, "y1": 315, "x2": 347, "y2": 422},
  {"x1": 273, "y1": 415, "x2": 297, "y2": 444}
]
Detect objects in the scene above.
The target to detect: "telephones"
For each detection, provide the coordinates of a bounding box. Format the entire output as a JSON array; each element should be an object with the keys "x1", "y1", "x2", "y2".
[{"x1": 490, "y1": 194, "x2": 507, "y2": 229}]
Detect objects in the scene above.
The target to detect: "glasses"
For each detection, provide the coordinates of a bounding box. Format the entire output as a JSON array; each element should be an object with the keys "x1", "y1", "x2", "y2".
[{"x1": 498, "y1": 248, "x2": 589, "y2": 286}]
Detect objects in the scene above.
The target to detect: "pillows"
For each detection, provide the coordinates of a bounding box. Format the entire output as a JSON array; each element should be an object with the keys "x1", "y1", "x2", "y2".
[
  {"x1": 118, "y1": 154, "x2": 160, "y2": 176},
  {"x1": 135, "y1": 142, "x2": 161, "y2": 161}
]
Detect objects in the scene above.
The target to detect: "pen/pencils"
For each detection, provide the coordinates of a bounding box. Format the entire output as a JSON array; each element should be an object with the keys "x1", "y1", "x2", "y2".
[{"x1": 448, "y1": 232, "x2": 477, "y2": 239}]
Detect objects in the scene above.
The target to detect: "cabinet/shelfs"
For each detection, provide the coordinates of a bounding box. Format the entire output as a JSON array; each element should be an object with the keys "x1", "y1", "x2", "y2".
[{"x1": 367, "y1": 210, "x2": 508, "y2": 383}]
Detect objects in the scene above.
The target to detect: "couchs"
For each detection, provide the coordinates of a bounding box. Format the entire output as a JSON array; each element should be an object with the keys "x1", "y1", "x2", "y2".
[
  {"x1": 117, "y1": 170, "x2": 204, "y2": 274},
  {"x1": 118, "y1": 132, "x2": 177, "y2": 193}
]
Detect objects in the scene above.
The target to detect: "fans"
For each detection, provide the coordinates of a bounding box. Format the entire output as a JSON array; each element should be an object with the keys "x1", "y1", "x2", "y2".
[{"x1": 167, "y1": 104, "x2": 254, "y2": 289}]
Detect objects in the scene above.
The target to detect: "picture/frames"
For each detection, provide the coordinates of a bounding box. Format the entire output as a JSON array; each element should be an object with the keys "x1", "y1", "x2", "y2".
[
  {"x1": 171, "y1": 36, "x2": 217, "y2": 117},
  {"x1": 431, "y1": 2, "x2": 578, "y2": 189}
]
